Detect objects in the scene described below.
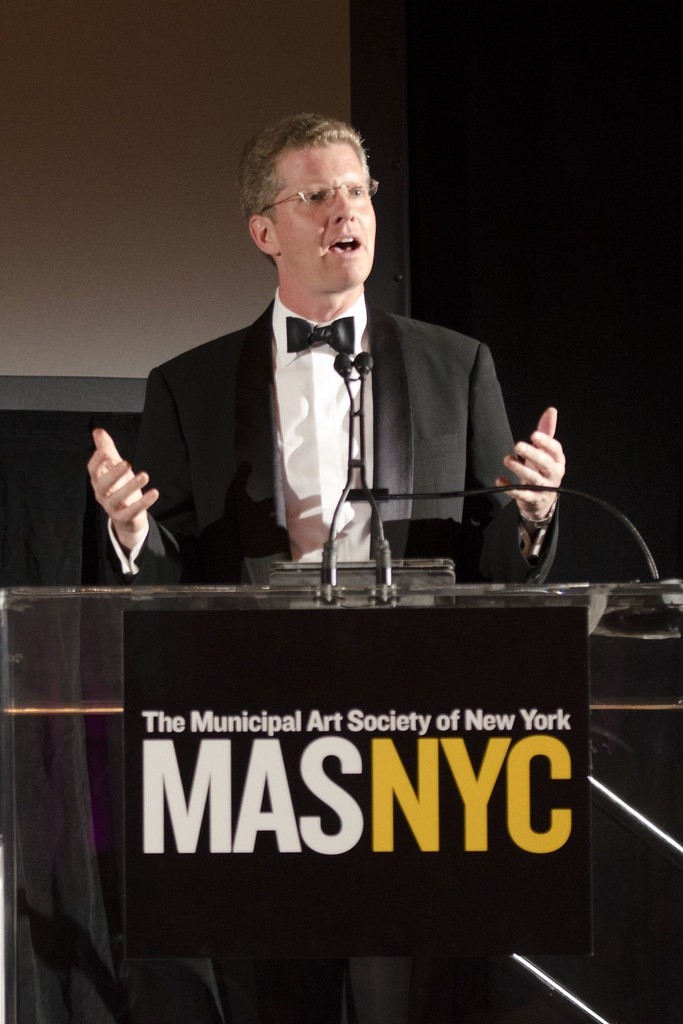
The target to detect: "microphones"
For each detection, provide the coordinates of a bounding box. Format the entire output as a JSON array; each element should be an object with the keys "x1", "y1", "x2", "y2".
[{"x1": 315, "y1": 352, "x2": 400, "y2": 604}]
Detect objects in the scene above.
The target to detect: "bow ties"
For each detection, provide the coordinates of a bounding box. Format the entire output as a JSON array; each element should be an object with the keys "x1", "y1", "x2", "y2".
[{"x1": 286, "y1": 316, "x2": 355, "y2": 356}]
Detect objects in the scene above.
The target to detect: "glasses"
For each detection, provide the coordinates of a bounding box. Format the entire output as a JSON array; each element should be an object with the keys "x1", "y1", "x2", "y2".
[{"x1": 260, "y1": 178, "x2": 379, "y2": 214}]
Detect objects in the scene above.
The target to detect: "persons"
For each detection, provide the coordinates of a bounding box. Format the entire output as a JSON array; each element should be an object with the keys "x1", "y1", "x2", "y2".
[{"x1": 88, "y1": 112, "x2": 565, "y2": 586}]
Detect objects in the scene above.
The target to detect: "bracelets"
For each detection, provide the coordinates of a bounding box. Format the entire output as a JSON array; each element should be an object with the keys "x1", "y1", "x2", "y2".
[{"x1": 518, "y1": 496, "x2": 557, "y2": 527}]
